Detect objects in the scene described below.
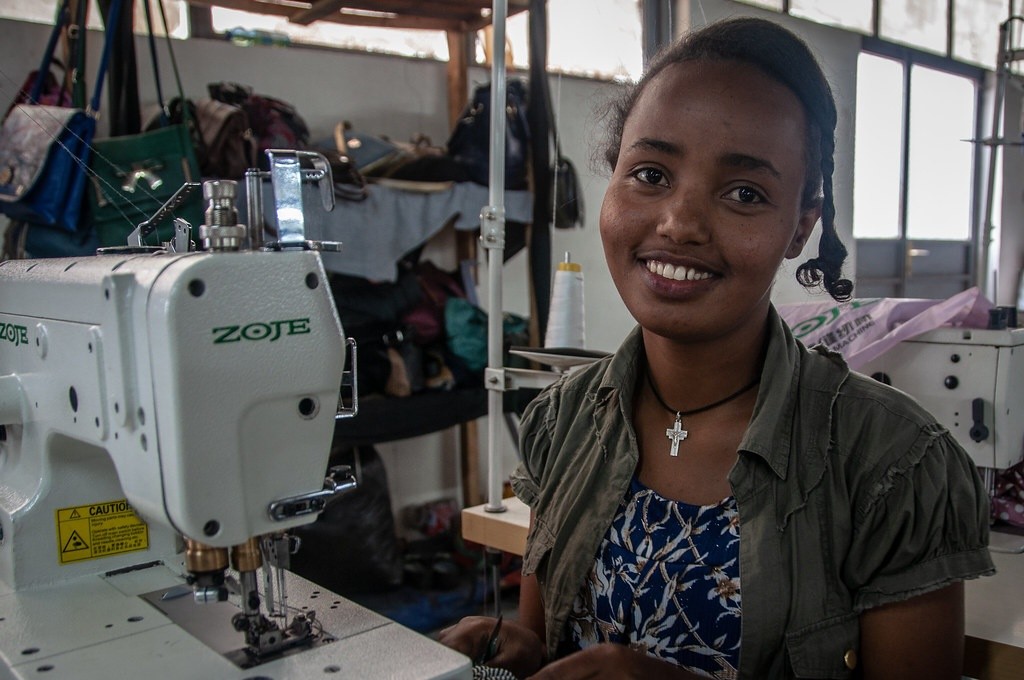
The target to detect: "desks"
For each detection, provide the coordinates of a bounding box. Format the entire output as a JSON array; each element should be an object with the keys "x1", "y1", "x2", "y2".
[{"x1": 461, "y1": 495, "x2": 1024, "y2": 649}]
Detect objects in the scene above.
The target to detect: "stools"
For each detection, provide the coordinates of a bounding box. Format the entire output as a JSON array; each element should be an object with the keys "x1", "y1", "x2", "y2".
[{"x1": 334, "y1": 379, "x2": 488, "y2": 596}]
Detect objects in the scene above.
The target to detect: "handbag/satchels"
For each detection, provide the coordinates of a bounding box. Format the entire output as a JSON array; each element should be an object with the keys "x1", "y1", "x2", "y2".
[
  {"x1": 0, "y1": 103, "x2": 95, "y2": 231},
  {"x1": 158, "y1": 69, "x2": 582, "y2": 401},
  {"x1": 1, "y1": 218, "x2": 88, "y2": 262},
  {"x1": 86, "y1": 124, "x2": 206, "y2": 251}
]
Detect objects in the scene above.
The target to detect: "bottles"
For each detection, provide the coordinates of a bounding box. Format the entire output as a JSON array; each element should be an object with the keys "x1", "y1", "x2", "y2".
[{"x1": 226, "y1": 27, "x2": 291, "y2": 48}]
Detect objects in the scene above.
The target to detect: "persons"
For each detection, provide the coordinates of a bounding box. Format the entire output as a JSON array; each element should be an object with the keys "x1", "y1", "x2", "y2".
[{"x1": 436, "y1": 15, "x2": 1000, "y2": 680}]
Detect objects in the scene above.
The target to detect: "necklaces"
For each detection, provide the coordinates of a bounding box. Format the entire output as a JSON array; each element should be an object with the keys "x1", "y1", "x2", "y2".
[{"x1": 646, "y1": 355, "x2": 763, "y2": 457}]
